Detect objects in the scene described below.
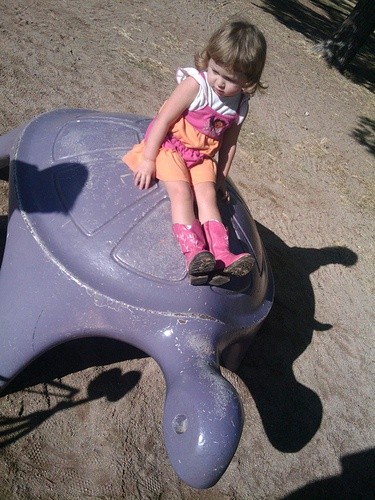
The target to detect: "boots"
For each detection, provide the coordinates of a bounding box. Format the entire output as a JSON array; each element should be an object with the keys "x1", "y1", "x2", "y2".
[
  {"x1": 202, "y1": 222, "x2": 255, "y2": 285},
  {"x1": 171, "y1": 220, "x2": 216, "y2": 286}
]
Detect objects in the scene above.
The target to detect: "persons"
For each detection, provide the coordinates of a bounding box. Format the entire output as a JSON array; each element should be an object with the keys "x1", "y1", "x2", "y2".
[{"x1": 122, "y1": 16, "x2": 270, "y2": 288}]
